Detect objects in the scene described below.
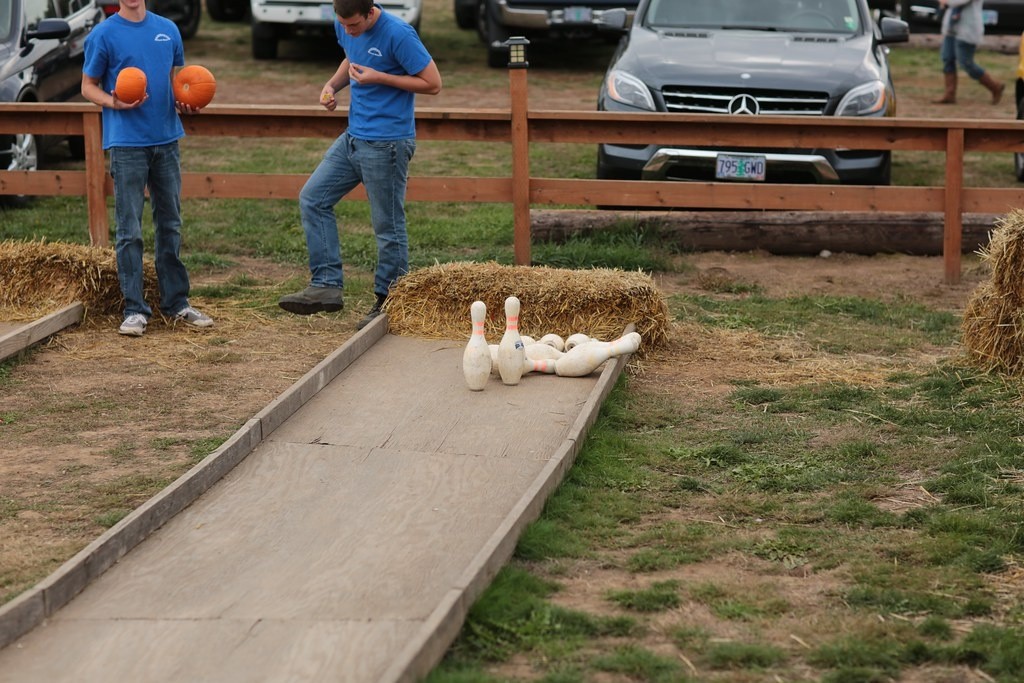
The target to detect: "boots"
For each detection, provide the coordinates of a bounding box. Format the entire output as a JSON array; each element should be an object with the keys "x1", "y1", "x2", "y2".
[
  {"x1": 930, "y1": 71, "x2": 957, "y2": 104},
  {"x1": 978, "y1": 71, "x2": 1006, "y2": 105}
]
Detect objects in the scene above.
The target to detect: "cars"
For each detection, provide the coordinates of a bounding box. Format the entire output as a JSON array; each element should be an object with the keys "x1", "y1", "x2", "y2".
[
  {"x1": 592, "y1": 0, "x2": 911, "y2": 199},
  {"x1": 863, "y1": 1, "x2": 1024, "y2": 39},
  {"x1": 252, "y1": 0, "x2": 424, "y2": 72},
  {"x1": 452, "y1": 0, "x2": 643, "y2": 75},
  {"x1": 0, "y1": 1, "x2": 202, "y2": 221}
]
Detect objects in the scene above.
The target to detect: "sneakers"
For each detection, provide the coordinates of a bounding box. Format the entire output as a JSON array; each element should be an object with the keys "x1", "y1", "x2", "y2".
[
  {"x1": 279, "y1": 286, "x2": 343, "y2": 313},
  {"x1": 175, "y1": 307, "x2": 214, "y2": 328},
  {"x1": 357, "y1": 296, "x2": 388, "y2": 330},
  {"x1": 118, "y1": 314, "x2": 147, "y2": 336}
]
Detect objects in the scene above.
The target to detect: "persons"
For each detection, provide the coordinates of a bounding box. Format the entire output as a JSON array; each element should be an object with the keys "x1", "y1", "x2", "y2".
[
  {"x1": 280, "y1": 0, "x2": 441, "y2": 328},
  {"x1": 929, "y1": 1, "x2": 1007, "y2": 107},
  {"x1": 79, "y1": 1, "x2": 216, "y2": 338}
]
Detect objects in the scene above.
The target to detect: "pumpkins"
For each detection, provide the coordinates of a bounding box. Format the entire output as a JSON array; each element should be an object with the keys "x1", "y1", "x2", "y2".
[
  {"x1": 173, "y1": 65, "x2": 216, "y2": 110},
  {"x1": 115, "y1": 67, "x2": 147, "y2": 104}
]
